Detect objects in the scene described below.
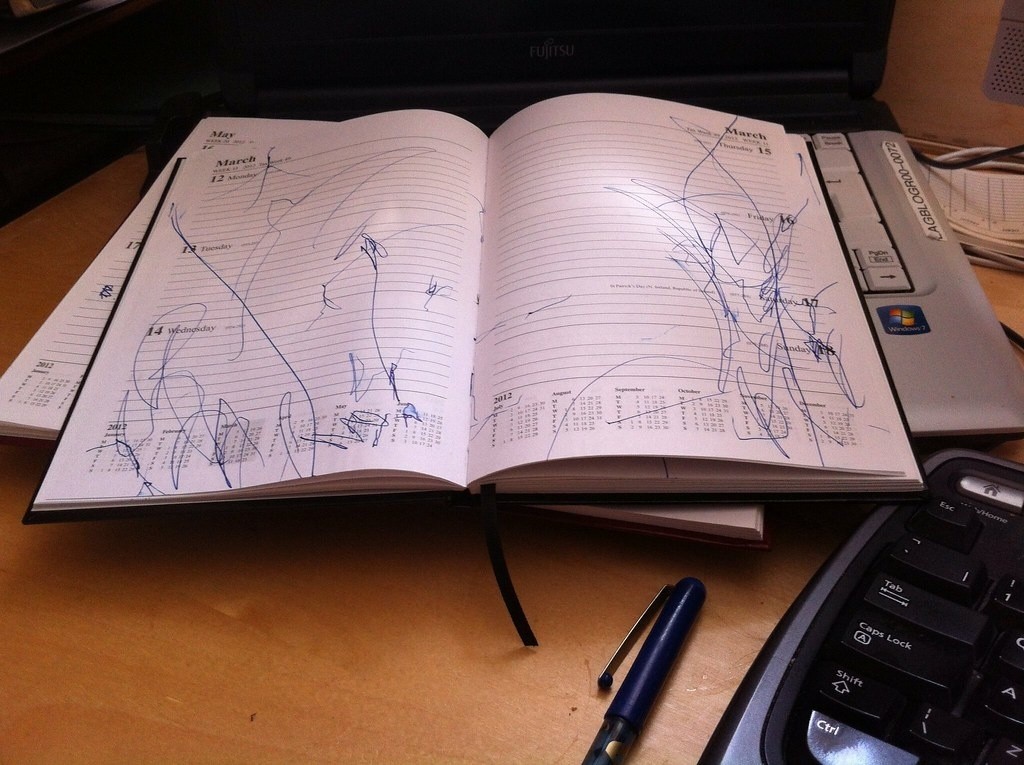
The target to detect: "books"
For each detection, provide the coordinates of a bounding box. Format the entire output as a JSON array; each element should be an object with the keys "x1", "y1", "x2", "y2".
[{"x1": 1, "y1": 92, "x2": 926, "y2": 554}]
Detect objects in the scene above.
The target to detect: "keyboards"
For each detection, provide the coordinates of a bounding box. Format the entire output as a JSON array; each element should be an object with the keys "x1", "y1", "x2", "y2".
[{"x1": 695, "y1": 448, "x2": 1024, "y2": 765}]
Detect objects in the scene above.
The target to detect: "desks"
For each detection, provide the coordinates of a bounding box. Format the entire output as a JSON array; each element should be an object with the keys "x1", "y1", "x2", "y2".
[{"x1": 0, "y1": 145, "x2": 1024, "y2": 765}]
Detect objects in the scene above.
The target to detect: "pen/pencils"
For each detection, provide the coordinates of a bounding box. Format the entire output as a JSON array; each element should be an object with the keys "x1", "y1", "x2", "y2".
[{"x1": 580, "y1": 575, "x2": 708, "y2": 764}]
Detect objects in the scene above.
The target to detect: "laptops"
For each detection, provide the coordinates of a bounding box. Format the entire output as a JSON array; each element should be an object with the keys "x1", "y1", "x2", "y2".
[{"x1": 140, "y1": 0, "x2": 1024, "y2": 442}]
